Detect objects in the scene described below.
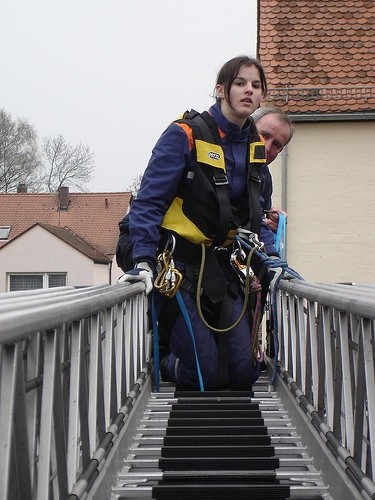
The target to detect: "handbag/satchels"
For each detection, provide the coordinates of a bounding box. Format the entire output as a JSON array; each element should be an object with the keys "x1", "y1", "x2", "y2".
[{"x1": 116, "y1": 209, "x2": 135, "y2": 272}]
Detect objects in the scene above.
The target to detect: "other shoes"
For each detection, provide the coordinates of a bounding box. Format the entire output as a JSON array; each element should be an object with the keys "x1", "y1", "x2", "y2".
[{"x1": 160, "y1": 356, "x2": 169, "y2": 381}]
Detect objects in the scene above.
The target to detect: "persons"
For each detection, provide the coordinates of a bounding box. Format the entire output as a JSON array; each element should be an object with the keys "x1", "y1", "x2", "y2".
[
  {"x1": 116, "y1": 55, "x2": 297, "y2": 385},
  {"x1": 251, "y1": 104, "x2": 294, "y2": 231}
]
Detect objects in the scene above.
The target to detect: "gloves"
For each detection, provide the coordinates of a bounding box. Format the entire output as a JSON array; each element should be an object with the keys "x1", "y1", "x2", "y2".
[
  {"x1": 267, "y1": 256, "x2": 286, "y2": 294},
  {"x1": 117, "y1": 261, "x2": 155, "y2": 295}
]
